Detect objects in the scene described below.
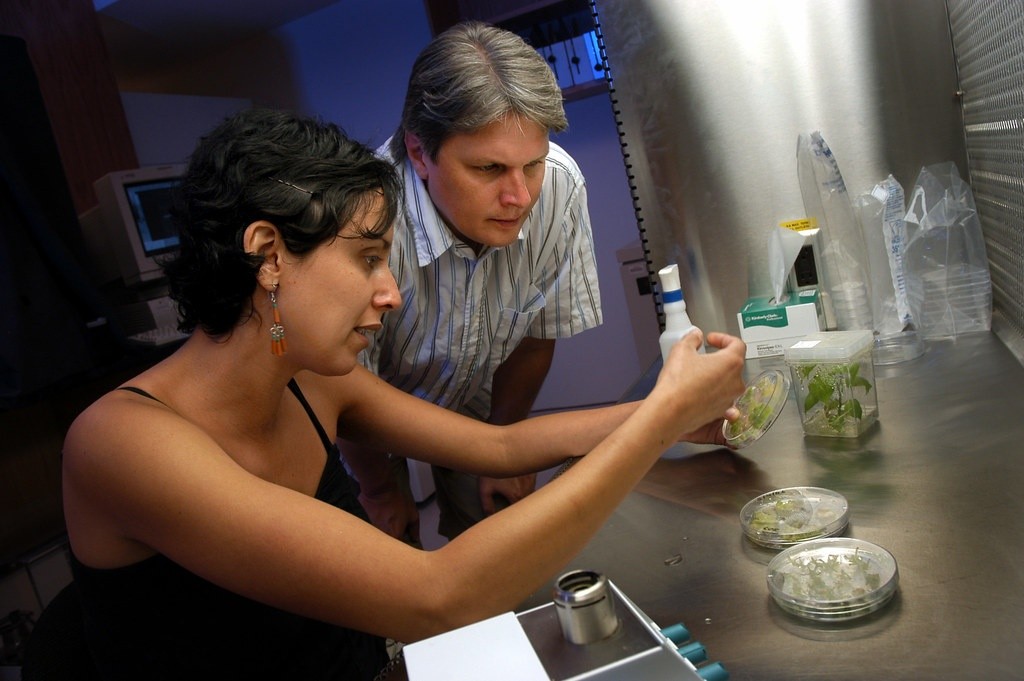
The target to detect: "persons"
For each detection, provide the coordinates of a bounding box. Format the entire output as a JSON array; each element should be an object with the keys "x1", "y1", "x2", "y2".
[
  {"x1": 334, "y1": 21, "x2": 603, "y2": 553},
  {"x1": 60, "y1": 109, "x2": 745, "y2": 681}
]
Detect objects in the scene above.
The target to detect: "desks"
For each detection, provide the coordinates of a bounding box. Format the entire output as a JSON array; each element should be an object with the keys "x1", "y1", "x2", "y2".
[{"x1": 514, "y1": 330, "x2": 1024, "y2": 681}]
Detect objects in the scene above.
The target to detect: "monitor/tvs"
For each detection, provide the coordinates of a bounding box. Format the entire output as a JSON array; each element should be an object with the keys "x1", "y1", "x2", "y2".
[{"x1": 78, "y1": 162, "x2": 189, "y2": 289}]
